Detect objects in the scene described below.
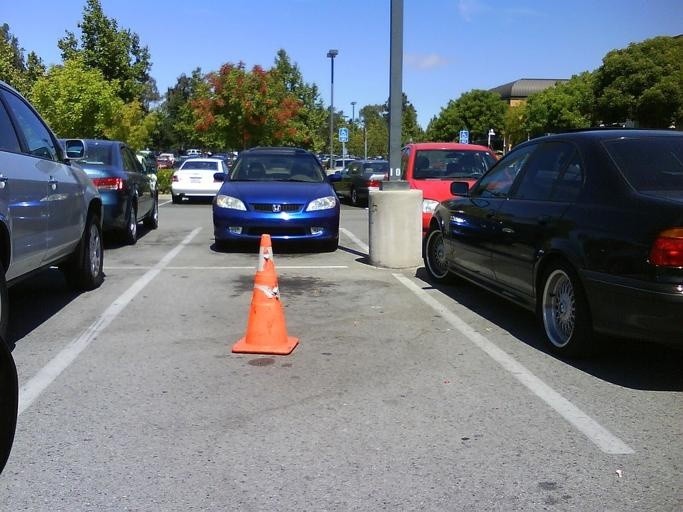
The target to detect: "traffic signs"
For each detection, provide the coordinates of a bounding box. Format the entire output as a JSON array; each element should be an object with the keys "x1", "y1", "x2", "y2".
[
  {"x1": 338, "y1": 128, "x2": 347, "y2": 142},
  {"x1": 459, "y1": 131, "x2": 468, "y2": 143}
]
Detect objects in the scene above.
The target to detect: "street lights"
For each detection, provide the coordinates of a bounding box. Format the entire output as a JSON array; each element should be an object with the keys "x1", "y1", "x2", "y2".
[
  {"x1": 327, "y1": 49, "x2": 338, "y2": 170},
  {"x1": 350, "y1": 102, "x2": 356, "y2": 139}
]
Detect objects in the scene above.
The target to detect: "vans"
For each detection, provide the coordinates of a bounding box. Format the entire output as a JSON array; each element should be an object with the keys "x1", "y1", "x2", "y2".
[{"x1": 324, "y1": 157, "x2": 356, "y2": 176}]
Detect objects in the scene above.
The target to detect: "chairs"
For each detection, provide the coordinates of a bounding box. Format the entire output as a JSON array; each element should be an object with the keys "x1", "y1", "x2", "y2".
[
  {"x1": 290, "y1": 162, "x2": 314, "y2": 179},
  {"x1": 248, "y1": 161, "x2": 265, "y2": 177},
  {"x1": 414, "y1": 155, "x2": 461, "y2": 177}
]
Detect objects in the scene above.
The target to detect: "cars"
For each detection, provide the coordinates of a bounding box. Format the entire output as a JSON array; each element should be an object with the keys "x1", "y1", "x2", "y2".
[
  {"x1": 157, "y1": 149, "x2": 238, "y2": 170},
  {"x1": 421, "y1": 129, "x2": 682, "y2": 359},
  {"x1": 0, "y1": 79, "x2": 103, "y2": 344},
  {"x1": 171, "y1": 157, "x2": 228, "y2": 205},
  {"x1": 57, "y1": 138, "x2": 159, "y2": 244},
  {"x1": 400, "y1": 142, "x2": 500, "y2": 238},
  {"x1": 212, "y1": 146, "x2": 340, "y2": 252},
  {"x1": 326, "y1": 160, "x2": 389, "y2": 206}
]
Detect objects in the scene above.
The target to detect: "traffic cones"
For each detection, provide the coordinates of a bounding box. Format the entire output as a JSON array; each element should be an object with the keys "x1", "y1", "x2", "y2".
[{"x1": 230, "y1": 232, "x2": 300, "y2": 356}]
